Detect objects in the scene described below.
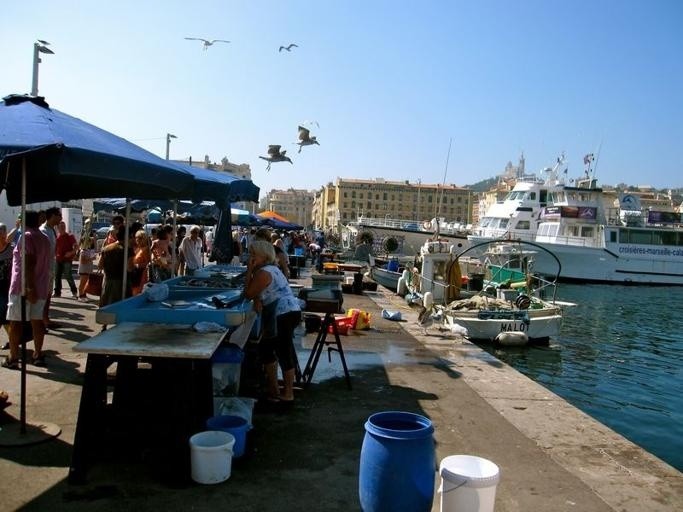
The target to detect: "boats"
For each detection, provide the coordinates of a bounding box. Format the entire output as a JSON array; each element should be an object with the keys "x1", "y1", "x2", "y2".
[
  {"x1": 377, "y1": 139, "x2": 565, "y2": 347},
  {"x1": 479, "y1": 240, "x2": 578, "y2": 312},
  {"x1": 370, "y1": 256, "x2": 404, "y2": 289}
]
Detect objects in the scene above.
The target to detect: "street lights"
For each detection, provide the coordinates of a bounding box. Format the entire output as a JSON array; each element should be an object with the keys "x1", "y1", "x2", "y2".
[
  {"x1": 30, "y1": 39, "x2": 56, "y2": 97},
  {"x1": 165, "y1": 133, "x2": 181, "y2": 158}
]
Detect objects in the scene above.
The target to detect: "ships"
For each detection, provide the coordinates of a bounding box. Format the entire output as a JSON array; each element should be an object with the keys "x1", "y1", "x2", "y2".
[
  {"x1": 466, "y1": 145, "x2": 683, "y2": 287},
  {"x1": 338, "y1": 212, "x2": 470, "y2": 258}
]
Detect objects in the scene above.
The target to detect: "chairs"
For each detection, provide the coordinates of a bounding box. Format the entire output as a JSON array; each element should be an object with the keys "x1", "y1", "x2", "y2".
[{"x1": 427, "y1": 216, "x2": 471, "y2": 236}]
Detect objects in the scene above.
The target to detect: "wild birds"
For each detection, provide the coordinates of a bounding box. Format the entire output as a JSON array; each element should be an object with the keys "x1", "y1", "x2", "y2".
[
  {"x1": 259, "y1": 145, "x2": 293, "y2": 173},
  {"x1": 420, "y1": 303, "x2": 437, "y2": 324},
  {"x1": 279, "y1": 44, "x2": 298, "y2": 52},
  {"x1": 293, "y1": 114, "x2": 320, "y2": 153},
  {"x1": 421, "y1": 316, "x2": 434, "y2": 335},
  {"x1": 183, "y1": 37, "x2": 230, "y2": 51}
]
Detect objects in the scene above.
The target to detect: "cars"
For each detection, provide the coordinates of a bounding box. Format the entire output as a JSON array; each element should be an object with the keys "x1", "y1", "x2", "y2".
[{"x1": 92, "y1": 226, "x2": 109, "y2": 239}]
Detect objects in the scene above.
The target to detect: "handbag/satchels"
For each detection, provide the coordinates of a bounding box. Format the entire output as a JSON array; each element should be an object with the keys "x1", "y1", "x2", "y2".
[
  {"x1": 85, "y1": 272, "x2": 104, "y2": 295},
  {"x1": 348, "y1": 308, "x2": 371, "y2": 330}
]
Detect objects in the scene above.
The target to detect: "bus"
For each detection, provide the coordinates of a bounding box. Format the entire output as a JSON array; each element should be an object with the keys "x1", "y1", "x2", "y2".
[{"x1": 141, "y1": 201, "x2": 248, "y2": 248}]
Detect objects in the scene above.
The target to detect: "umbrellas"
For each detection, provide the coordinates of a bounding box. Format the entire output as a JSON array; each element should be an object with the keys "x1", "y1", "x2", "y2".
[
  {"x1": 122, "y1": 157, "x2": 261, "y2": 301},
  {"x1": 0, "y1": 93, "x2": 196, "y2": 446}
]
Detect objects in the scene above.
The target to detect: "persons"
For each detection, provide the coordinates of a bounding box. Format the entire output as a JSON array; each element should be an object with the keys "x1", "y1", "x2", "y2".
[
  {"x1": 77, "y1": 218, "x2": 98, "y2": 303},
  {"x1": 3, "y1": 207, "x2": 62, "y2": 365},
  {"x1": 240, "y1": 239, "x2": 303, "y2": 404},
  {"x1": 55, "y1": 221, "x2": 78, "y2": 300},
  {"x1": 99, "y1": 215, "x2": 321, "y2": 306}
]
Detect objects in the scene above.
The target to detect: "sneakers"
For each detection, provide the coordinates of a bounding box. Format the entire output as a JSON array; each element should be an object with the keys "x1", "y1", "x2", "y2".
[
  {"x1": 1, "y1": 356, "x2": 19, "y2": 369},
  {"x1": 26, "y1": 351, "x2": 44, "y2": 366}
]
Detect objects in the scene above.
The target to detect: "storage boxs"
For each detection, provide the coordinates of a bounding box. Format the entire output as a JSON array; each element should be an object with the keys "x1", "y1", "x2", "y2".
[
  {"x1": 494, "y1": 287, "x2": 517, "y2": 302},
  {"x1": 305, "y1": 297, "x2": 340, "y2": 313},
  {"x1": 345, "y1": 276, "x2": 353, "y2": 285}
]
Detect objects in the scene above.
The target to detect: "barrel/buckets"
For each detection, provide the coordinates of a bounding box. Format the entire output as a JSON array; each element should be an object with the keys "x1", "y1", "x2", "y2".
[
  {"x1": 358, "y1": 411, "x2": 436, "y2": 511},
  {"x1": 207, "y1": 415, "x2": 249, "y2": 458},
  {"x1": 436, "y1": 454, "x2": 500, "y2": 512},
  {"x1": 188, "y1": 430, "x2": 236, "y2": 485}
]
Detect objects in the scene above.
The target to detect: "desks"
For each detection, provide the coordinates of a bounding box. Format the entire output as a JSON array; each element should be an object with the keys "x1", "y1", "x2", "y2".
[
  {"x1": 302, "y1": 307, "x2": 353, "y2": 393},
  {"x1": 287, "y1": 254, "x2": 304, "y2": 278},
  {"x1": 68, "y1": 322, "x2": 231, "y2": 483},
  {"x1": 288, "y1": 284, "x2": 303, "y2": 297},
  {"x1": 336, "y1": 263, "x2": 362, "y2": 292}
]
